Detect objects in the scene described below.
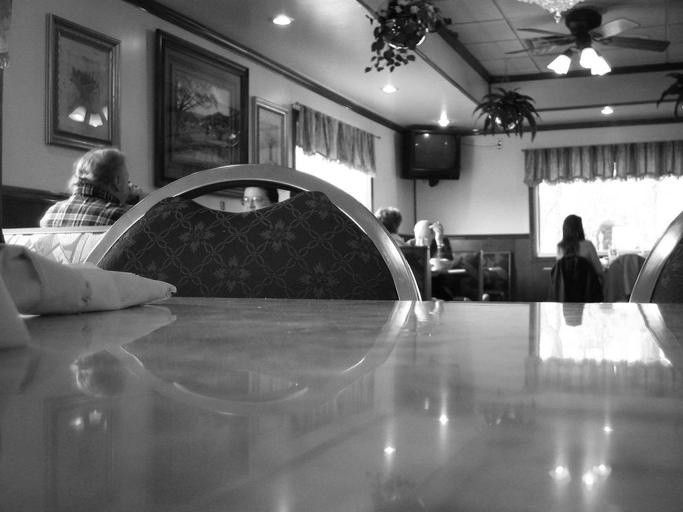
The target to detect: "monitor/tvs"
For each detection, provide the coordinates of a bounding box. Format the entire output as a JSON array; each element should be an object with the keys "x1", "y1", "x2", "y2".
[{"x1": 400, "y1": 128, "x2": 461, "y2": 180}]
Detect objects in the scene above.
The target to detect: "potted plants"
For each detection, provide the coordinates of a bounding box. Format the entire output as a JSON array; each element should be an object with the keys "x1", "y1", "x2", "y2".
[
  {"x1": 472, "y1": 86, "x2": 540, "y2": 142},
  {"x1": 361, "y1": 1, "x2": 452, "y2": 75}
]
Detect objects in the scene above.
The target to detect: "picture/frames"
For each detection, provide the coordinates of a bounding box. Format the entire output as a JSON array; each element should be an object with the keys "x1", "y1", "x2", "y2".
[
  {"x1": 42, "y1": 9, "x2": 124, "y2": 153},
  {"x1": 152, "y1": 21, "x2": 295, "y2": 193}
]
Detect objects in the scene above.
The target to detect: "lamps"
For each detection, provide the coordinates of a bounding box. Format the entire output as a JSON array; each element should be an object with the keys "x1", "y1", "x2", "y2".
[{"x1": 547, "y1": 38, "x2": 611, "y2": 79}]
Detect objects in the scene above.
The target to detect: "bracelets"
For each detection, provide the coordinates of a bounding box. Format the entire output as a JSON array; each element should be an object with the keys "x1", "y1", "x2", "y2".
[{"x1": 435, "y1": 242, "x2": 446, "y2": 248}]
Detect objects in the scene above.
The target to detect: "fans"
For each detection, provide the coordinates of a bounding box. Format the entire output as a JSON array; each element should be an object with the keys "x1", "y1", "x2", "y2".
[{"x1": 503, "y1": 8, "x2": 672, "y2": 62}]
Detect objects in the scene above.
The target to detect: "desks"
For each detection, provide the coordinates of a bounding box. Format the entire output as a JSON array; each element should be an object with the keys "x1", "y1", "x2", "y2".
[{"x1": 0, "y1": 284, "x2": 681, "y2": 512}]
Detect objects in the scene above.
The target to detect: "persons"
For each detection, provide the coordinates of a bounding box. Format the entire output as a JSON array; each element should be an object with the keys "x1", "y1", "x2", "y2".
[
  {"x1": 37, "y1": 146, "x2": 149, "y2": 229},
  {"x1": 555, "y1": 213, "x2": 606, "y2": 283},
  {"x1": 374, "y1": 207, "x2": 452, "y2": 301},
  {"x1": 239, "y1": 185, "x2": 280, "y2": 213},
  {"x1": 404, "y1": 220, "x2": 489, "y2": 300}
]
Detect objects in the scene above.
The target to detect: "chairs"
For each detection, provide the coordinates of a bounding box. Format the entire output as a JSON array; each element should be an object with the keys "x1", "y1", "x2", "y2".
[
  {"x1": 545, "y1": 209, "x2": 682, "y2": 303},
  {"x1": 82, "y1": 163, "x2": 423, "y2": 303}
]
